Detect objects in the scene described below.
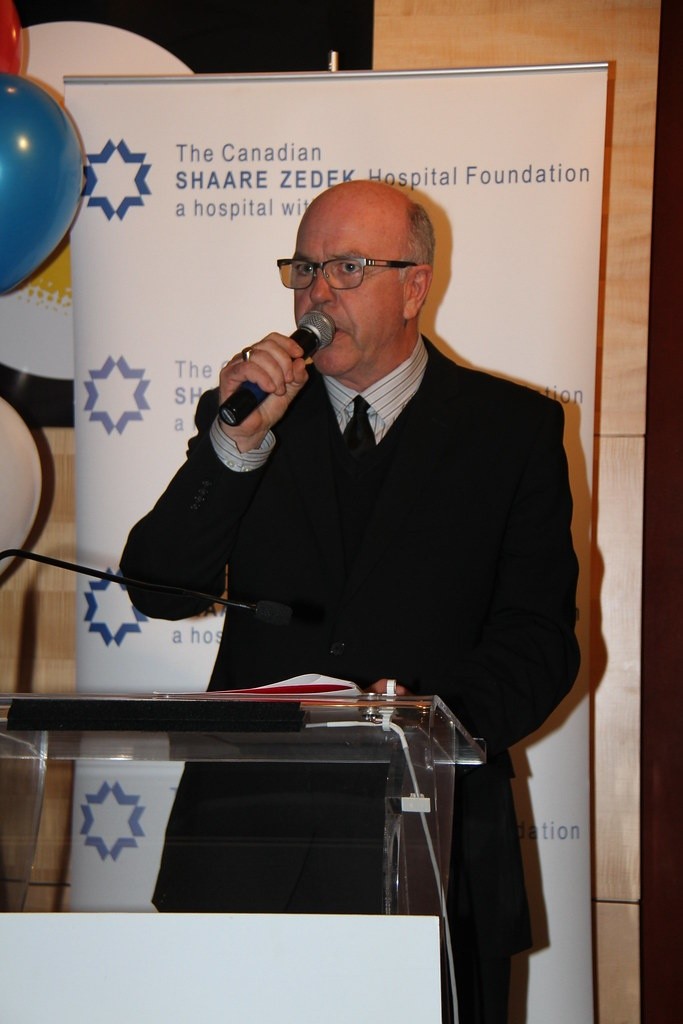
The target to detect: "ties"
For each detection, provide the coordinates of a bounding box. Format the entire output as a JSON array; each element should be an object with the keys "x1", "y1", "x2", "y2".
[{"x1": 343, "y1": 395, "x2": 375, "y2": 462}]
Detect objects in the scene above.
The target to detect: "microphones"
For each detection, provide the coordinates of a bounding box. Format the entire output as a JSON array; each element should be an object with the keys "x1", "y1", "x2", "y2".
[
  {"x1": 219, "y1": 310, "x2": 334, "y2": 426},
  {"x1": 0, "y1": 547, "x2": 292, "y2": 629}
]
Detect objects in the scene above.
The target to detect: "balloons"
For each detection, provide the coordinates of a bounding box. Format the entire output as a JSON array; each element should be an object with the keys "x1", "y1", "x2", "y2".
[
  {"x1": 0, "y1": 0, "x2": 84, "y2": 296},
  {"x1": 1, "y1": 398, "x2": 41, "y2": 573}
]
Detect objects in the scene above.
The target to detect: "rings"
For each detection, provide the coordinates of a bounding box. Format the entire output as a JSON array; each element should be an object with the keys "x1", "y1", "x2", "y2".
[{"x1": 243, "y1": 344, "x2": 252, "y2": 363}]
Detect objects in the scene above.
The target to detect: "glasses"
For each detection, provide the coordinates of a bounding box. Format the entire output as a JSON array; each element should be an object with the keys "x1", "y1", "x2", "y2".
[{"x1": 277, "y1": 257, "x2": 417, "y2": 290}]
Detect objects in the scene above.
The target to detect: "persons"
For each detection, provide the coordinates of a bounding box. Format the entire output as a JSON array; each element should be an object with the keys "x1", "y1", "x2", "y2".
[{"x1": 119, "y1": 179, "x2": 583, "y2": 1024}]
